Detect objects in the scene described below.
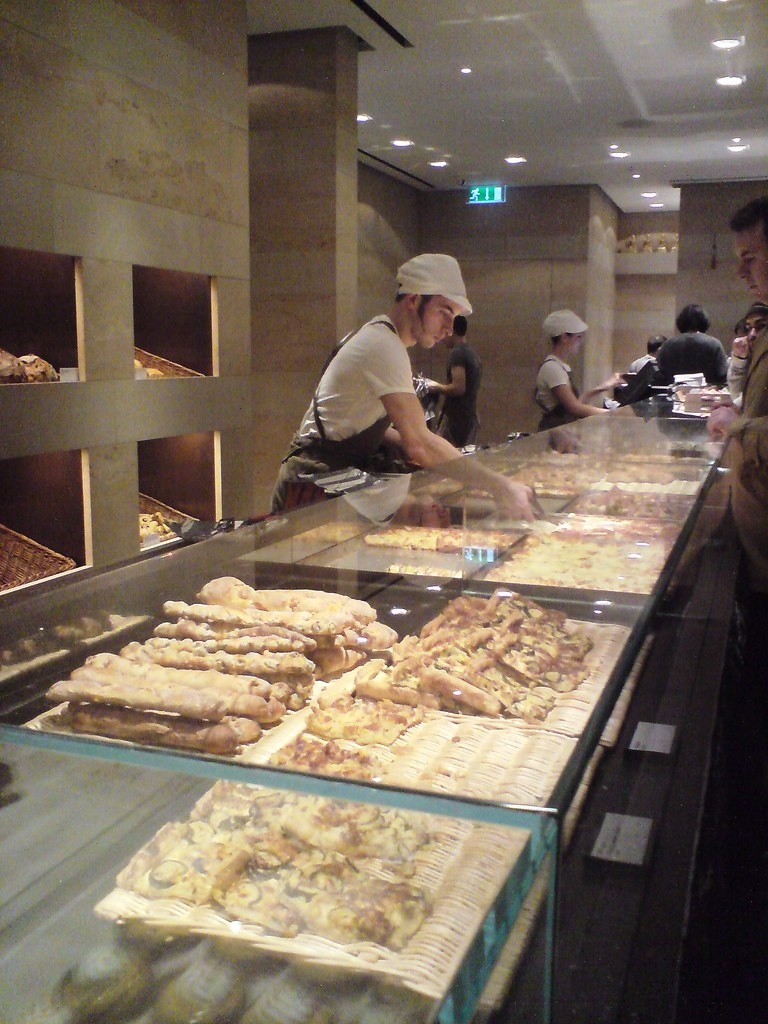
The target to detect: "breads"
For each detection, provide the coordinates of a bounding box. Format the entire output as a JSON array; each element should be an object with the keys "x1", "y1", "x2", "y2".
[
  {"x1": 140, "y1": 511, "x2": 179, "y2": 546},
  {"x1": 0, "y1": 348, "x2": 59, "y2": 384},
  {"x1": 45, "y1": 578, "x2": 399, "y2": 752},
  {"x1": 134, "y1": 360, "x2": 165, "y2": 379}
]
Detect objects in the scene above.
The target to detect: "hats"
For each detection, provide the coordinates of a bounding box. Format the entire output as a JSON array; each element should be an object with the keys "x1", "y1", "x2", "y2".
[
  {"x1": 543, "y1": 309, "x2": 588, "y2": 336},
  {"x1": 397, "y1": 253, "x2": 473, "y2": 317},
  {"x1": 734, "y1": 301, "x2": 768, "y2": 337}
]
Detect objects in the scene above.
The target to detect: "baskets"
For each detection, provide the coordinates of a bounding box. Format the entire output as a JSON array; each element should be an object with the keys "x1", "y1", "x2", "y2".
[
  {"x1": 139, "y1": 493, "x2": 199, "y2": 548},
  {"x1": 134, "y1": 348, "x2": 204, "y2": 378},
  {"x1": 0, "y1": 525, "x2": 76, "y2": 592}
]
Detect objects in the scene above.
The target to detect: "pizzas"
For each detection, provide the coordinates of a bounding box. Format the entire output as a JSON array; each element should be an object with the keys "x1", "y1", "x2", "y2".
[
  {"x1": 365, "y1": 454, "x2": 708, "y2": 594},
  {"x1": 114, "y1": 584, "x2": 594, "y2": 950}
]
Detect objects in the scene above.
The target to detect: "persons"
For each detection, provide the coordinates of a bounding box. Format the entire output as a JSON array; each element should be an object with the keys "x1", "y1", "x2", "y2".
[
  {"x1": 344, "y1": 473, "x2": 466, "y2": 588},
  {"x1": 272, "y1": 254, "x2": 537, "y2": 519},
  {"x1": 426, "y1": 315, "x2": 480, "y2": 449},
  {"x1": 658, "y1": 303, "x2": 728, "y2": 384},
  {"x1": 704, "y1": 195, "x2": 768, "y2": 595},
  {"x1": 628, "y1": 335, "x2": 667, "y2": 373},
  {"x1": 534, "y1": 309, "x2": 625, "y2": 433}
]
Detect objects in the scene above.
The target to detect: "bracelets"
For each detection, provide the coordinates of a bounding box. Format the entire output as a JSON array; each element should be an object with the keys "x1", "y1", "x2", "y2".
[{"x1": 734, "y1": 355, "x2": 748, "y2": 360}]
